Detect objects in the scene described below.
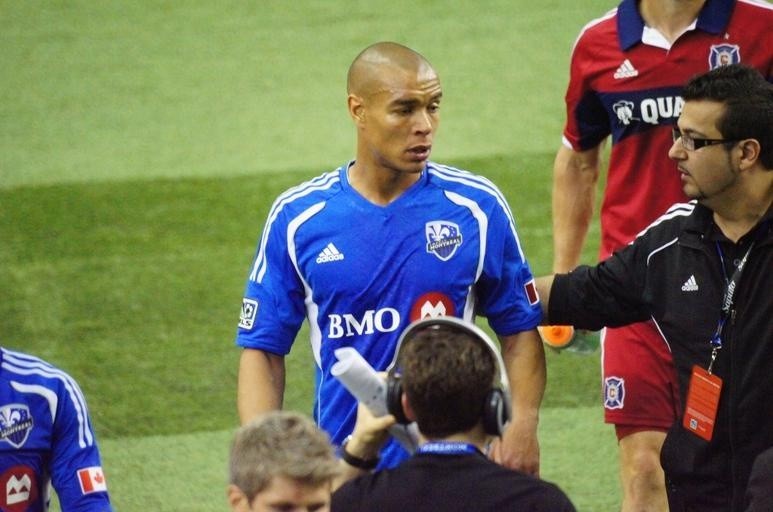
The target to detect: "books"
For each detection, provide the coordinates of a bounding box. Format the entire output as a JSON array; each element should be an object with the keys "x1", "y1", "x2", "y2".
[{"x1": 332, "y1": 344, "x2": 423, "y2": 458}]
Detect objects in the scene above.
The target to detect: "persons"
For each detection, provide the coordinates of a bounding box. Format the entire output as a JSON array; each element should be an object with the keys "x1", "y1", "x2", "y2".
[
  {"x1": 237, "y1": 43, "x2": 546, "y2": 479},
  {"x1": 225, "y1": 411, "x2": 338, "y2": 511},
  {"x1": 552, "y1": 0, "x2": 773, "y2": 512},
  {"x1": 474, "y1": 65, "x2": 772, "y2": 511},
  {"x1": 746, "y1": 446, "x2": 773, "y2": 510},
  {"x1": 1, "y1": 349, "x2": 113, "y2": 511},
  {"x1": 329, "y1": 321, "x2": 577, "y2": 510}
]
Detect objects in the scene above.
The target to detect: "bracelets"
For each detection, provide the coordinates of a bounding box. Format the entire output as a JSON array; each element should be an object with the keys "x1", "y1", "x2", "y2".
[{"x1": 339, "y1": 433, "x2": 379, "y2": 469}]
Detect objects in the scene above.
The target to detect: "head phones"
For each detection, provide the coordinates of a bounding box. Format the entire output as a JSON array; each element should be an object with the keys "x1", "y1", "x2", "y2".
[{"x1": 384, "y1": 312, "x2": 514, "y2": 438}]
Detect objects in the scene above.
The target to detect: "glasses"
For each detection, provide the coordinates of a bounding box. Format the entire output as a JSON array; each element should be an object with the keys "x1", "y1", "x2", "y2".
[{"x1": 672, "y1": 127, "x2": 731, "y2": 151}]
